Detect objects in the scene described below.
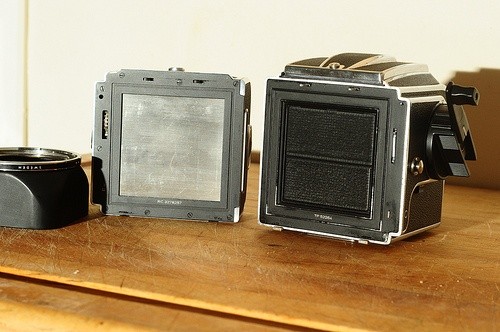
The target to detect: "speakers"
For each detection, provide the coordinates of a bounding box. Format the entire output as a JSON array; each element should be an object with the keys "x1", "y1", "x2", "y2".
[
  {"x1": 91, "y1": 70, "x2": 253, "y2": 223},
  {"x1": 257, "y1": 54, "x2": 477, "y2": 244}
]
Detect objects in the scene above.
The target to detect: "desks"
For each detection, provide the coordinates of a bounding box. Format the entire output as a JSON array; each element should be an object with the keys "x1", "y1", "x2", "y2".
[{"x1": 0, "y1": 160, "x2": 499, "y2": 332}]
